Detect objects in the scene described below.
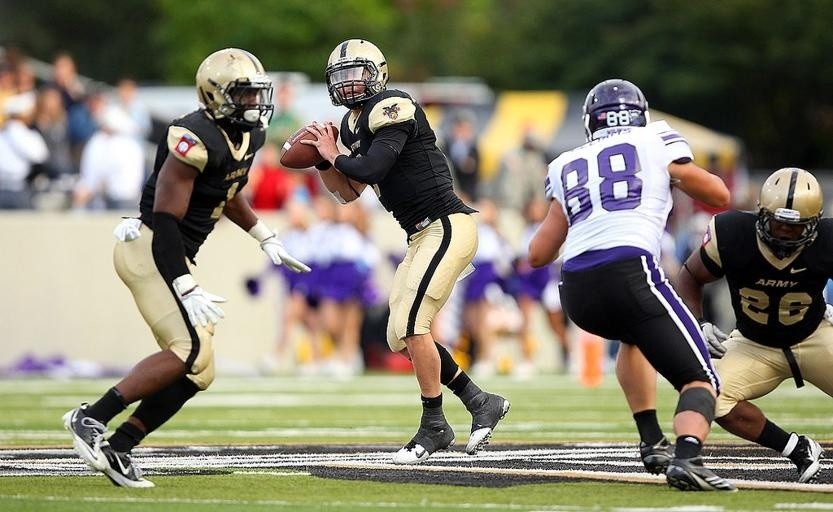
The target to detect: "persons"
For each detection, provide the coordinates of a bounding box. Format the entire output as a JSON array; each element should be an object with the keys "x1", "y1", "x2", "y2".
[
  {"x1": 527, "y1": 77, "x2": 739, "y2": 494},
  {"x1": 580, "y1": 328, "x2": 622, "y2": 389},
  {"x1": 62, "y1": 46, "x2": 314, "y2": 490},
  {"x1": 1, "y1": 42, "x2": 153, "y2": 212},
  {"x1": 439, "y1": 111, "x2": 480, "y2": 207},
  {"x1": 241, "y1": 187, "x2": 400, "y2": 380},
  {"x1": 690, "y1": 151, "x2": 737, "y2": 340},
  {"x1": 452, "y1": 196, "x2": 574, "y2": 387},
  {"x1": 299, "y1": 38, "x2": 511, "y2": 466},
  {"x1": 676, "y1": 165, "x2": 833, "y2": 485},
  {"x1": 264, "y1": 79, "x2": 303, "y2": 153}
]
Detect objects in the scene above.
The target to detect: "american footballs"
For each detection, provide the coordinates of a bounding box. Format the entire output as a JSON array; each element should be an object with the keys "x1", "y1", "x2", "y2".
[{"x1": 279, "y1": 122, "x2": 340, "y2": 169}]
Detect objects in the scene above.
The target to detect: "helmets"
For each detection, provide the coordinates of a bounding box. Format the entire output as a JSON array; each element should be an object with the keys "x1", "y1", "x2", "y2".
[
  {"x1": 196, "y1": 48, "x2": 275, "y2": 128},
  {"x1": 754, "y1": 168, "x2": 824, "y2": 258},
  {"x1": 582, "y1": 80, "x2": 651, "y2": 141},
  {"x1": 325, "y1": 40, "x2": 390, "y2": 108}
]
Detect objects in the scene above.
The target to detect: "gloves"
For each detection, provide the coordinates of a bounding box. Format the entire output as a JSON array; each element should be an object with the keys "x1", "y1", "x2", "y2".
[
  {"x1": 699, "y1": 321, "x2": 730, "y2": 359},
  {"x1": 262, "y1": 237, "x2": 313, "y2": 274},
  {"x1": 179, "y1": 288, "x2": 228, "y2": 328}
]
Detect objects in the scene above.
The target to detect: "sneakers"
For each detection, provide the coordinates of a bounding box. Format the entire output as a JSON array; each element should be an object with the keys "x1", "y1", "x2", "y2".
[
  {"x1": 466, "y1": 390, "x2": 511, "y2": 452},
  {"x1": 665, "y1": 453, "x2": 741, "y2": 494},
  {"x1": 96, "y1": 439, "x2": 156, "y2": 488},
  {"x1": 63, "y1": 408, "x2": 106, "y2": 469},
  {"x1": 781, "y1": 432, "x2": 825, "y2": 484},
  {"x1": 638, "y1": 436, "x2": 678, "y2": 476},
  {"x1": 393, "y1": 414, "x2": 457, "y2": 465}
]
